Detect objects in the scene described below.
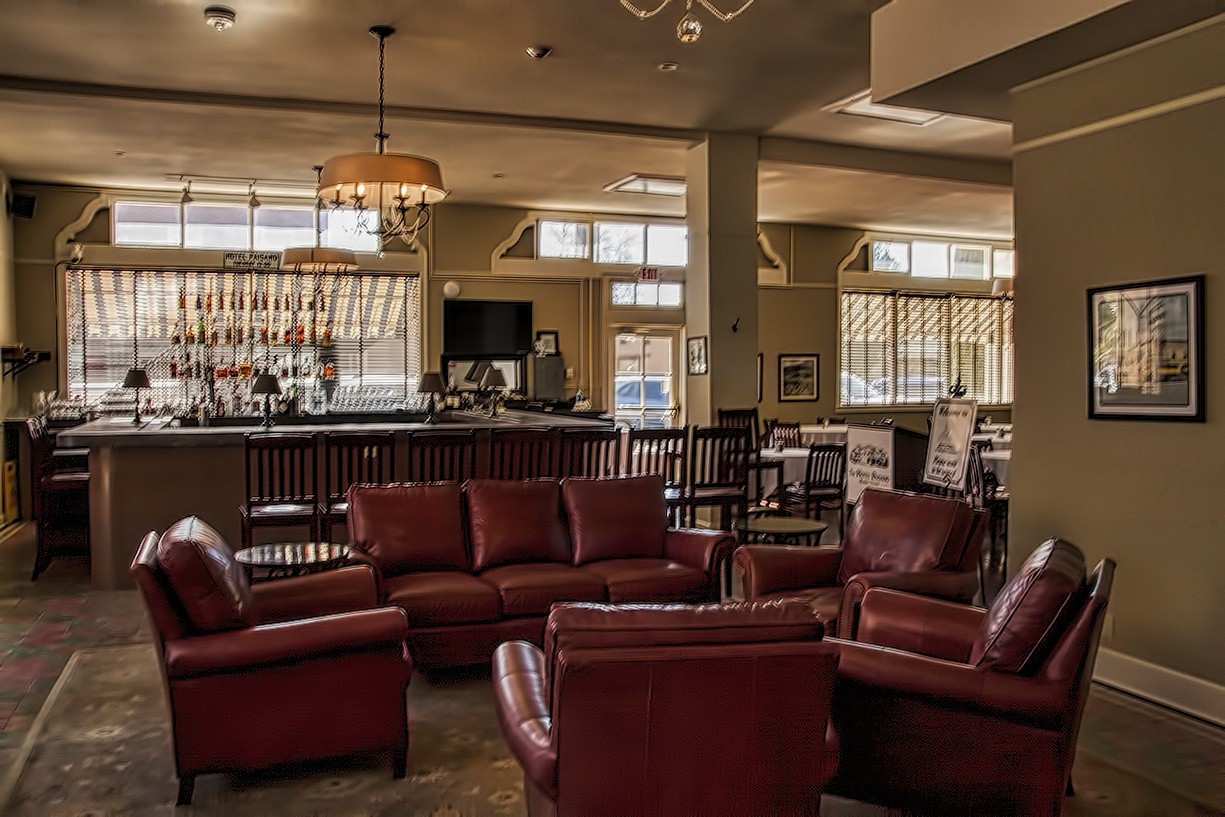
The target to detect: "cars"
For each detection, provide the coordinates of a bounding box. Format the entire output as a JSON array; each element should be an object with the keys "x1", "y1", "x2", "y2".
[
  {"x1": 616, "y1": 371, "x2": 672, "y2": 429},
  {"x1": 838, "y1": 368, "x2": 952, "y2": 403}
]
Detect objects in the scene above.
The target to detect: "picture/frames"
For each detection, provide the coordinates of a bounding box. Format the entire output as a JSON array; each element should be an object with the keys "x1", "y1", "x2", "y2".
[
  {"x1": 778, "y1": 353, "x2": 820, "y2": 403},
  {"x1": 756, "y1": 353, "x2": 763, "y2": 403},
  {"x1": 687, "y1": 335, "x2": 708, "y2": 376},
  {"x1": 537, "y1": 331, "x2": 560, "y2": 358},
  {"x1": 1086, "y1": 272, "x2": 1206, "y2": 423}
]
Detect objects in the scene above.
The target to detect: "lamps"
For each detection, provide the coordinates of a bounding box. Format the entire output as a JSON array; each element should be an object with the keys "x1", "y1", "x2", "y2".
[
  {"x1": 473, "y1": 362, "x2": 493, "y2": 401},
  {"x1": 317, "y1": 24, "x2": 450, "y2": 238},
  {"x1": 482, "y1": 369, "x2": 509, "y2": 420},
  {"x1": 125, "y1": 367, "x2": 153, "y2": 423},
  {"x1": 420, "y1": 373, "x2": 448, "y2": 423},
  {"x1": 253, "y1": 373, "x2": 284, "y2": 430},
  {"x1": 619, "y1": 0, "x2": 756, "y2": 44}
]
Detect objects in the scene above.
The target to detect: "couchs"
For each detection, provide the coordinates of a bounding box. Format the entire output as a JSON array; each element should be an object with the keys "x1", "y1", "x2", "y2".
[{"x1": 129, "y1": 474, "x2": 1116, "y2": 817}]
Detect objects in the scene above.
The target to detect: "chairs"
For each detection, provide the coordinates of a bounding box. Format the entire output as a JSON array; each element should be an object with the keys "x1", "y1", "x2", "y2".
[
  {"x1": 241, "y1": 408, "x2": 849, "y2": 531},
  {"x1": 966, "y1": 446, "x2": 1007, "y2": 560},
  {"x1": 23, "y1": 414, "x2": 91, "y2": 581}
]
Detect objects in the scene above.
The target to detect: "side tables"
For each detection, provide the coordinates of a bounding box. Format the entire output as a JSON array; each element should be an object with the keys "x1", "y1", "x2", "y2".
[
  {"x1": 233, "y1": 540, "x2": 350, "y2": 583},
  {"x1": 734, "y1": 517, "x2": 830, "y2": 546}
]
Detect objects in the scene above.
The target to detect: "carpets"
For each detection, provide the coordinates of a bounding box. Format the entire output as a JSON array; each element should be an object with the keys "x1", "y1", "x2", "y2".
[{"x1": 0, "y1": 643, "x2": 1222, "y2": 817}]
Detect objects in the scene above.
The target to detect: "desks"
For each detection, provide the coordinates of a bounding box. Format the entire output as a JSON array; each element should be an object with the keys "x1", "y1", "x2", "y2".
[
  {"x1": 970, "y1": 419, "x2": 1014, "y2": 496},
  {"x1": 747, "y1": 450, "x2": 847, "y2": 501},
  {"x1": 769, "y1": 424, "x2": 850, "y2": 449}
]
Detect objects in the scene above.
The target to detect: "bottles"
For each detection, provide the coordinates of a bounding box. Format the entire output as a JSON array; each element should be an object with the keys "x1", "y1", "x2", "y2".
[{"x1": 144, "y1": 294, "x2": 335, "y2": 418}]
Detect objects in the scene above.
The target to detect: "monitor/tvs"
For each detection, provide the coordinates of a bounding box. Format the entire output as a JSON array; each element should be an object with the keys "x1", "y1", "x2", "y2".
[{"x1": 442, "y1": 298, "x2": 533, "y2": 356}]
